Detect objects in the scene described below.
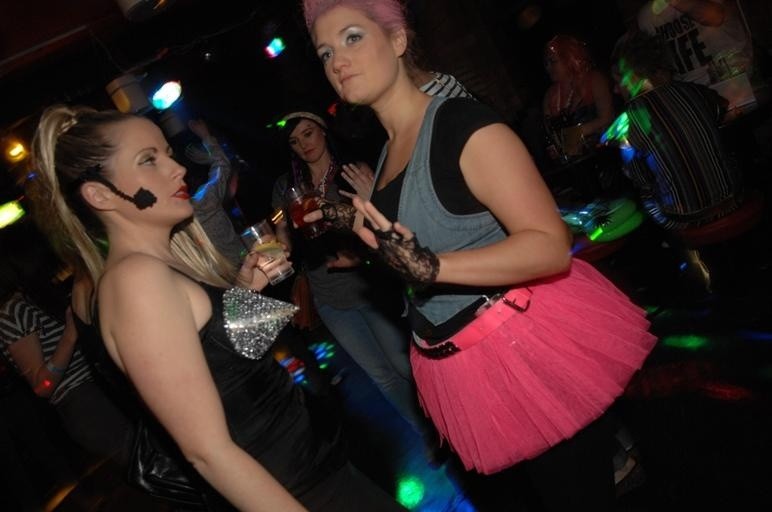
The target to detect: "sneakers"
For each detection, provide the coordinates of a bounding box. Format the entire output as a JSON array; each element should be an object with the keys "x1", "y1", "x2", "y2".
[{"x1": 612, "y1": 438, "x2": 658, "y2": 500}]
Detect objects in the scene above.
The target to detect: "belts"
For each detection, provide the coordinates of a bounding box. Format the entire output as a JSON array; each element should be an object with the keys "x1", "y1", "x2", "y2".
[{"x1": 410, "y1": 278, "x2": 536, "y2": 361}]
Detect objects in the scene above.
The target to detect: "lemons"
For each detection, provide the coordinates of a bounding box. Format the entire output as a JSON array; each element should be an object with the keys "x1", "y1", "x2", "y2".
[{"x1": 253, "y1": 241, "x2": 284, "y2": 256}]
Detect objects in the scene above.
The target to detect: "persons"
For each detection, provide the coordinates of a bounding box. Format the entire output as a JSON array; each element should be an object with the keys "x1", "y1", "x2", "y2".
[{"x1": 1, "y1": 0, "x2": 772, "y2": 512}]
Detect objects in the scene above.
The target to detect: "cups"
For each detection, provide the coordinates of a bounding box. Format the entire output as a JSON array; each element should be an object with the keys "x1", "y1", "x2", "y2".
[
  {"x1": 239, "y1": 218, "x2": 296, "y2": 285},
  {"x1": 281, "y1": 182, "x2": 327, "y2": 240}
]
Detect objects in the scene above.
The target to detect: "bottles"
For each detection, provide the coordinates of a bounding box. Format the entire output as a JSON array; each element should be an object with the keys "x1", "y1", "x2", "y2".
[{"x1": 539, "y1": 127, "x2": 559, "y2": 160}]
